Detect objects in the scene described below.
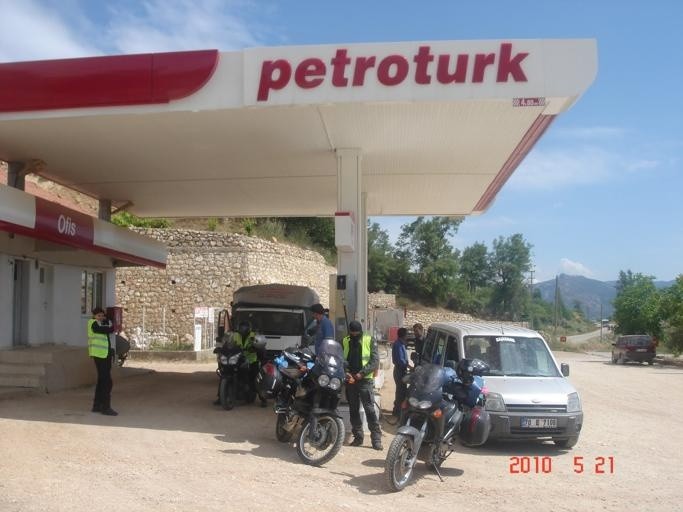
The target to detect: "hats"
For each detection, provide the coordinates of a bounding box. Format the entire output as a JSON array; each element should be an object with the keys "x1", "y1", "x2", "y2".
[
  {"x1": 349, "y1": 320, "x2": 363, "y2": 331},
  {"x1": 309, "y1": 303, "x2": 325, "y2": 315}
]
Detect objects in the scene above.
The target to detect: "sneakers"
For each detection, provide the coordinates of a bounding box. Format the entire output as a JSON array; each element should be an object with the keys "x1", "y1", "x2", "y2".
[
  {"x1": 348, "y1": 436, "x2": 365, "y2": 447},
  {"x1": 370, "y1": 439, "x2": 385, "y2": 450}
]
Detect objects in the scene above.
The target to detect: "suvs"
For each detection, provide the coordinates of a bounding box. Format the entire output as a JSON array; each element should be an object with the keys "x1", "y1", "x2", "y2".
[{"x1": 611, "y1": 335, "x2": 656, "y2": 365}]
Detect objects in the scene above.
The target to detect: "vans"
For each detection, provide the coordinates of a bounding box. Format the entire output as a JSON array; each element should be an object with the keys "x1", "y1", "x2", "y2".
[{"x1": 421, "y1": 323, "x2": 582, "y2": 448}]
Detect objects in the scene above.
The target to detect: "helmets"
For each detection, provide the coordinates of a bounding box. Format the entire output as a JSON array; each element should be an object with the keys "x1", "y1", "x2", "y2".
[{"x1": 457, "y1": 356, "x2": 490, "y2": 386}]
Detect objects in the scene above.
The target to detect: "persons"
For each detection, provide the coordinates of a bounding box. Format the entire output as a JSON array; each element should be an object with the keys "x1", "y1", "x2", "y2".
[
  {"x1": 391, "y1": 327, "x2": 414, "y2": 419},
  {"x1": 339, "y1": 320, "x2": 386, "y2": 452},
  {"x1": 86, "y1": 306, "x2": 119, "y2": 416},
  {"x1": 411, "y1": 322, "x2": 427, "y2": 355},
  {"x1": 307, "y1": 303, "x2": 341, "y2": 413},
  {"x1": 212, "y1": 319, "x2": 268, "y2": 407}
]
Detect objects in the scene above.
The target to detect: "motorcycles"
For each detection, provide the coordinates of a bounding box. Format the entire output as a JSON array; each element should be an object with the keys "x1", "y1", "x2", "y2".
[
  {"x1": 273, "y1": 337, "x2": 345, "y2": 465},
  {"x1": 214, "y1": 343, "x2": 257, "y2": 410},
  {"x1": 384, "y1": 356, "x2": 473, "y2": 490}
]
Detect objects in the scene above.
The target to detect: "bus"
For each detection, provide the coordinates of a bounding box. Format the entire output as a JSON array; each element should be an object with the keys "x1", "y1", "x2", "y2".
[{"x1": 602, "y1": 319, "x2": 608, "y2": 327}]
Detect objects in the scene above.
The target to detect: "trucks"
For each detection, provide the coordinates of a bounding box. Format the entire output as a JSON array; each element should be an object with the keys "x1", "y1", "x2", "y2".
[{"x1": 230, "y1": 284, "x2": 320, "y2": 362}]
